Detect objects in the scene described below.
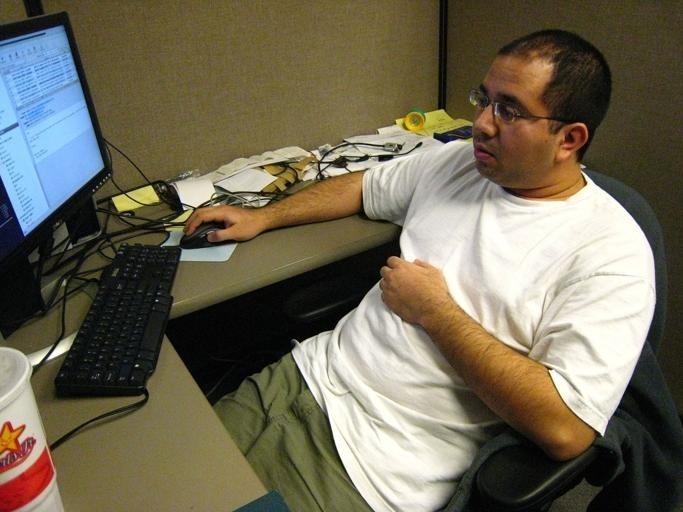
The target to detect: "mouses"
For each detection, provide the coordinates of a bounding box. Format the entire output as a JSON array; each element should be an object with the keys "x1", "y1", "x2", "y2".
[{"x1": 180, "y1": 220, "x2": 237, "y2": 249}]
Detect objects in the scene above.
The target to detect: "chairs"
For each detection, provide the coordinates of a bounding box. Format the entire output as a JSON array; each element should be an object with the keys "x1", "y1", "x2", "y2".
[{"x1": 409, "y1": 163, "x2": 666, "y2": 510}]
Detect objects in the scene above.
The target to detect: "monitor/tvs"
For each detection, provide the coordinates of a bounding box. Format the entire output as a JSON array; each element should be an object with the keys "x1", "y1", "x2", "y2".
[{"x1": 0, "y1": 10, "x2": 112, "y2": 276}]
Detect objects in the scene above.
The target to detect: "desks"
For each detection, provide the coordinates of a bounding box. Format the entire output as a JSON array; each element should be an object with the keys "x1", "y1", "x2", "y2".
[{"x1": 1, "y1": 145, "x2": 400, "y2": 511}]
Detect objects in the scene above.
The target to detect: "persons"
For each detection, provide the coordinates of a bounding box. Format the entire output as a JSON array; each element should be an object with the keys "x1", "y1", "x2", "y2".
[{"x1": 182, "y1": 30, "x2": 657, "y2": 512}]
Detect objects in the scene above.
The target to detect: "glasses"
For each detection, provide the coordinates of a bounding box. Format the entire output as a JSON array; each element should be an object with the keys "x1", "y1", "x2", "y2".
[{"x1": 469, "y1": 88, "x2": 571, "y2": 124}]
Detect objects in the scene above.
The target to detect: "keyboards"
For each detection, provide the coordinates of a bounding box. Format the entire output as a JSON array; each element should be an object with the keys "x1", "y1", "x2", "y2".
[{"x1": 53, "y1": 242, "x2": 182, "y2": 398}]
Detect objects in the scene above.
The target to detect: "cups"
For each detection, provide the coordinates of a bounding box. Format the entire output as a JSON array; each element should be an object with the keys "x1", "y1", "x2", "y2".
[{"x1": 0, "y1": 349, "x2": 63, "y2": 511}]
